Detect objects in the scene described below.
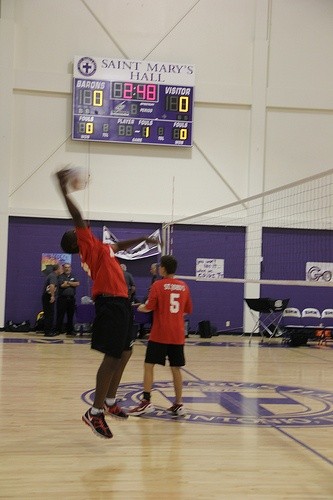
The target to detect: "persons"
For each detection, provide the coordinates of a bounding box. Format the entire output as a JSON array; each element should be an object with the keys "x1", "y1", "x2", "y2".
[
  {"x1": 150, "y1": 263, "x2": 160, "y2": 283},
  {"x1": 43, "y1": 264, "x2": 63, "y2": 337},
  {"x1": 54, "y1": 263, "x2": 80, "y2": 336},
  {"x1": 124, "y1": 255, "x2": 193, "y2": 416},
  {"x1": 120, "y1": 264, "x2": 136, "y2": 347},
  {"x1": 56, "y1": 168, "x2": 161, "y2": 440}
]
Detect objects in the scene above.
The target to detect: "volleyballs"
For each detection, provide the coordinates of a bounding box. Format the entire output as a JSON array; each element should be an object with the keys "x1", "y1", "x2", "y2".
[{"x1": 70, "y1": 167, "x2": 89, "y2": 190}]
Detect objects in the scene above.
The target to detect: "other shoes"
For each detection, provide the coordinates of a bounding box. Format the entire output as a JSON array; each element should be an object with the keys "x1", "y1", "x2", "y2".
[{"x1": 44, "y1": 328, "x2": 78, "y2": 337}]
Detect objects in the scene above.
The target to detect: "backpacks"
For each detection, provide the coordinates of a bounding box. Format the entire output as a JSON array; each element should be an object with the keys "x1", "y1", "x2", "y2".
[{"x1": 35, "y1": 312, "x2": 44, "y2": 331}]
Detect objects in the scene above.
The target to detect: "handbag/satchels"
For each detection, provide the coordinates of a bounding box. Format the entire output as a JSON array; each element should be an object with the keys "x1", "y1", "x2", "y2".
[{"x1": 5, "y1": 319, "x2": 30, "y2": 333}]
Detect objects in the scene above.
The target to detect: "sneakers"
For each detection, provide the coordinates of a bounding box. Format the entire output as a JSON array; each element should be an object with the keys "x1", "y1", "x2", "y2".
[
  {"x1": 82, "y1": 408, "x2": 113, "y2": 439},
  {"x1": 166, "y1": 404, "x2": 186, "y2": 416},
  {"x1": 128, "y1": 400, "x2": 155, "y2": 415},
  {"x1": 104, "y1": 401, "x2": 129, "y2": 419}
]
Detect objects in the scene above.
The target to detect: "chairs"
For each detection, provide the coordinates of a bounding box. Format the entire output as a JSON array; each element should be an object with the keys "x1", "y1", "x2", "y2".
[{"x1": 243, "y1": 297, "x2": 333, "y2": 345}]
